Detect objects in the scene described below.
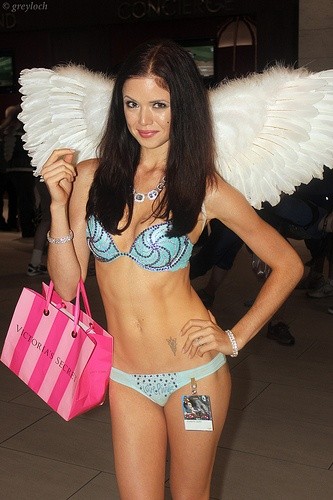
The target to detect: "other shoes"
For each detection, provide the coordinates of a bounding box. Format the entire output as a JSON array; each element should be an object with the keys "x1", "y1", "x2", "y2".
[
  {"x1": 244, "y1": 300, "x2": 254, "y2": 307},
  {"x1": 256, "y1": 271, "x2": 266, "y2": 282},
  {"x1": 294, "y1": 275, "x2": 325, "y2": 289},
  {"x1": 328, "y1": 306, "x2": 333, "y2": 313},
  {"x1": 196, "y1": 287, "x2": 216, "y2": 307},
  {"x1": 0, "y1": 217, "x2": 18, "y2": 232},
  {"x1": 304, "y1": 259, "x2": 312, "y2": 266},
  {"x1": 267, "y1": 321, "x2": 295, "y2": 346},
  {"x1": 306, "y1": 281, "x2": 333, "y2": 297}
]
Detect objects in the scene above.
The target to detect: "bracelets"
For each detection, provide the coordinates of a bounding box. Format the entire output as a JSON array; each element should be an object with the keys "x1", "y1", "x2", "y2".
[
  {"x1": 226, "y1": 330, "x2": 238, "y2": 358},
  {"x1": 47, "y1": 229, "x2": 74, "y2": 244}
]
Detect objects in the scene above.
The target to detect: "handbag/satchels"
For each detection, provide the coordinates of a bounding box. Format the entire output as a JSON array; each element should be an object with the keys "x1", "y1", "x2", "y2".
[{"x1": 0, "y1": 277, "x2": 113, "y2": 421}]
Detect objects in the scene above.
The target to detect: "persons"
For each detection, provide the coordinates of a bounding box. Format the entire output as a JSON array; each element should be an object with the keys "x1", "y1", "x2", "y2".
[
  {"x1": 189, "y1": 165, "x2": 333, "y2": 347},
  {"x1": 41, "y1": 40, "x2": 304, "y2": 500},
  {"x1": 0, "y1": 106, "x2": 52, "y2": 275}
]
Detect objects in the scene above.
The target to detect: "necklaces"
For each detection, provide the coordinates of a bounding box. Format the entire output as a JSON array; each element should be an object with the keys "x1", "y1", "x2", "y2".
[{"x1": 133, "y1": 175, "x2": 165, "y2": 202}]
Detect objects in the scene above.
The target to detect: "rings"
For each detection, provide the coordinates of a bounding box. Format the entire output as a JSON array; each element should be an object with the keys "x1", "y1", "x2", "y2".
[{"x1": 196, "y1": 339, "x2": 201, "y2": 347}]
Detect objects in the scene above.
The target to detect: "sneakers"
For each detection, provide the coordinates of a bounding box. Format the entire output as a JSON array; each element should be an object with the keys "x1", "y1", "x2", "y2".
[{"x1": 27, "y1": 263, "x2": 47, "y2": 276}]
